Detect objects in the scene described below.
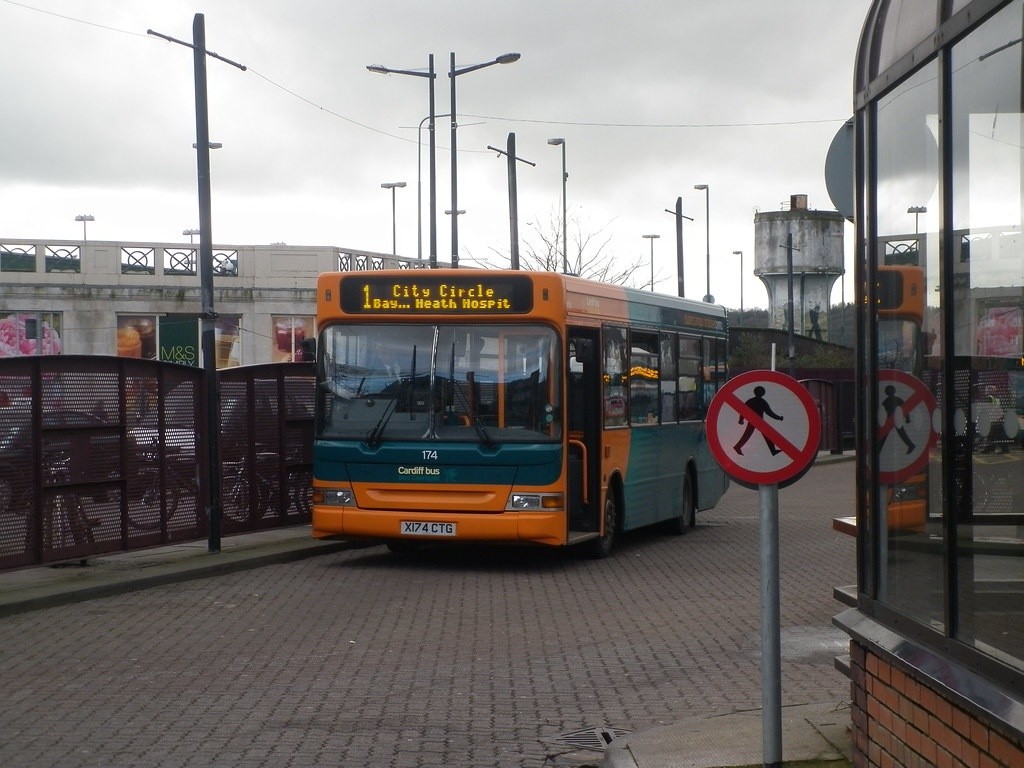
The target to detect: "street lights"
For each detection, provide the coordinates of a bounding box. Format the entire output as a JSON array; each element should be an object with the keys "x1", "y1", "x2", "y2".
[
  {"x1": 548, "y1": 137, "x2": 569, "y2": 274},
  {"x1": 448, "y1": 51, "x2": 521, "y2": 268},
  {"x1": 380, "y1": 182, "x2": 406, "y2": 256},
  {"x1": 694, "y1": 183, "x2": 712, "y2": 303},
  {"x1": 906, "y1": 206, "x2": 928, "y2": 237},
  {"x1": 183, "y1": 229, "x2": 200, "y2": 273},
  {"x1": 642, "y1": 234, "x2": 660, "y2": 293},
  {"x1": 74, "y1": 215, "x2": 95, "y2": 245},
  {"x1": 733, "y1": 250, "x2": 744, "y2": 328},
  {"x1": 366, "y1": 53, "x2": 438, "y2": 269}
]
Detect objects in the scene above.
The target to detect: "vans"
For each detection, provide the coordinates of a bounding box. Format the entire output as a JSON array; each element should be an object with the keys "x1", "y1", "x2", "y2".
[{"x1": 124, "y1": 379, "x2": 318, "y2": 479}]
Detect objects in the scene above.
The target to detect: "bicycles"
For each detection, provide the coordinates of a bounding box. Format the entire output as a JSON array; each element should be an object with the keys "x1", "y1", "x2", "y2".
[
  {"x1": 16, "y1": 450, "x2": 104, "y2": 567},
  {"x1": 117, "y1": 436, "x2": 312, "y2": 528}
]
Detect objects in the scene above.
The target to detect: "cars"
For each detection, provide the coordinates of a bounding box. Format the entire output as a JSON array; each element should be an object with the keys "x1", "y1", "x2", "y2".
[{"x1": 0, "y1": 407, "x2": 137, "y2": 514}]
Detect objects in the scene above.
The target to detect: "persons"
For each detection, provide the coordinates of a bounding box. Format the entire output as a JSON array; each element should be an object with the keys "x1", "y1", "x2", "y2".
[
  {"x1": 369, "y1": 343, "x2": 412, "y2": 397},
  {"x1": 88, "y1": 400, "x2": 110, "y2": 423},
  {"x1": 980, "y1": 384, "x2": 1011, "y2": 456}
]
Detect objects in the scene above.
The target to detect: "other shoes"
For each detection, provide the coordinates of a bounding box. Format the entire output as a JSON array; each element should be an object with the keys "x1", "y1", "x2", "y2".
[
  {"x1": 981, "y1": 450, "x2": 993, "y2": 454},
  {"x1": 997, "y1": 450, "x2": 1009, "y2": 454}
]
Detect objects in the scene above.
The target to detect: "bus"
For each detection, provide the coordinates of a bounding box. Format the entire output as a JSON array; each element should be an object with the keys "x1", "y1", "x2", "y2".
[{"x1": 302, "y1": 269, "x2": 737, "y2": 559}]
